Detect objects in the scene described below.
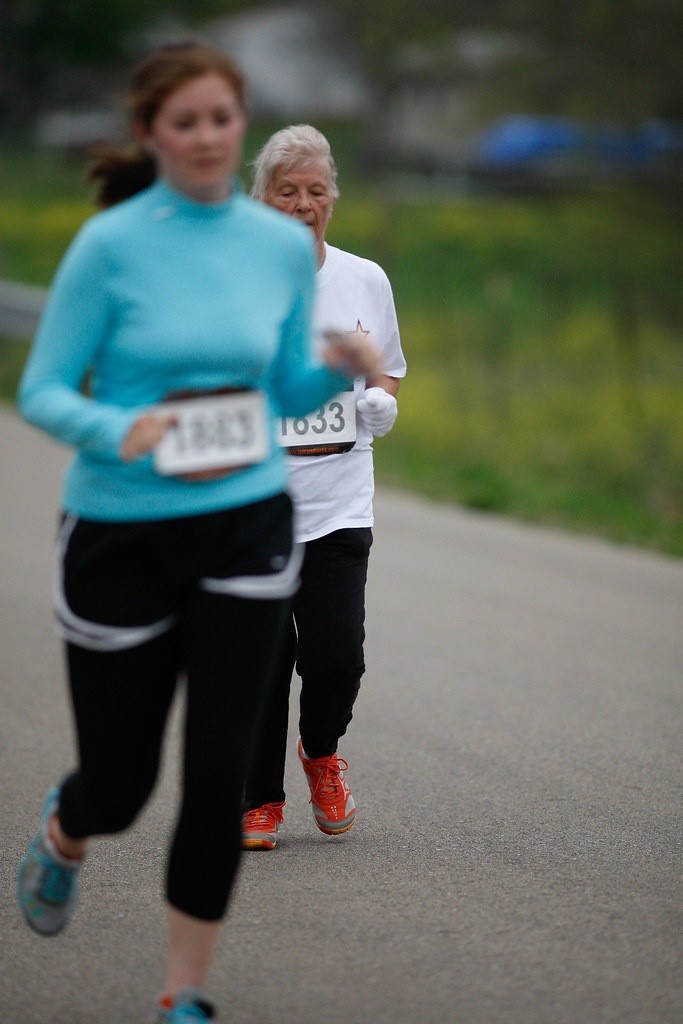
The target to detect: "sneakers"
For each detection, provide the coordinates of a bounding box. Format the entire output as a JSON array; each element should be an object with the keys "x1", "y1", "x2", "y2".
[
  {"x1": 17, "y1": 787, "x2": 87, "y2": 937},
  {"x1": 155, "y1": 986, "x2": 215, "y2": 1024},
  {"x1": 241, "y1": 801, "x2": 286, "y2": 849},
  {"x1": 295, "y1": 735, "x2": 357, "y2": 835}
]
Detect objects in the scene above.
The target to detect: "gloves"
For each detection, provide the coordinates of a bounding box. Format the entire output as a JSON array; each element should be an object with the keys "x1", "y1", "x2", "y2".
[{"x1": 356, "y1": 387, "x2": 398, "y2": 439}]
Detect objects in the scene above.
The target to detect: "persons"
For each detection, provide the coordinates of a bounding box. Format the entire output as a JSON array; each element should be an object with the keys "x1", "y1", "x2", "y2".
[
  {"x1": 16, "y1": 39, "x2": 386, "y2": 1024},
  {"x1": 242, "y1": 124, "x2": 408, "y2": 850}
]
